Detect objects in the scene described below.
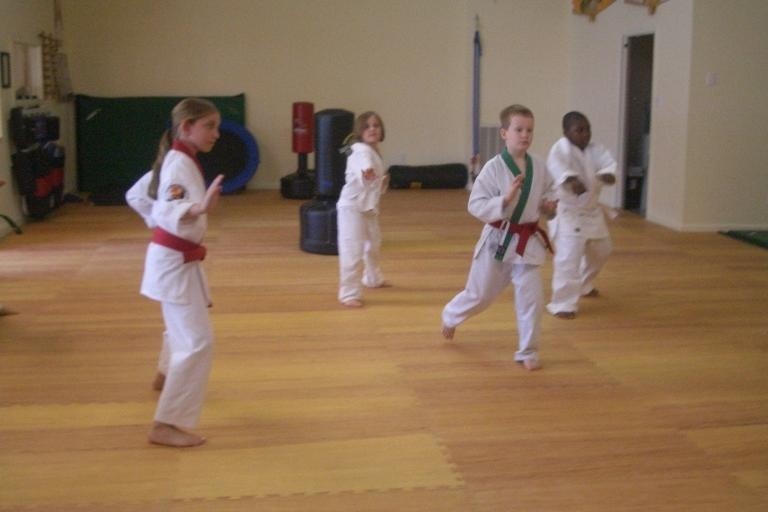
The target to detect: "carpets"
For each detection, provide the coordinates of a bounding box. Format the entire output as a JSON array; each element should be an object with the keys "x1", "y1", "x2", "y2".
[{"x1": 719, "y1": 229, "x2": 768, "y2": 250}]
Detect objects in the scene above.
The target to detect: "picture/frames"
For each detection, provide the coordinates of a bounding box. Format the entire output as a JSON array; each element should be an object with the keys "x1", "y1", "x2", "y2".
[{"x1": 0, "y1": 53, "x2": 10, "y2": 87}]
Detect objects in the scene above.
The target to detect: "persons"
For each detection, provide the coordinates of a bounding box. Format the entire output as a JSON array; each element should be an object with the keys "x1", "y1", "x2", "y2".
[
  {"x1": 125, "y1": 98, "x2": 224, "y2": 446},
  {"x1": 335, "y1": 112, "x2": 392, "y2": 308},
  {"x1": 441, "y1": 105, "x2": 559, "y2": 371},
  {"x1": 545, "y1": 110, "x2": 617, "y2": 319}
]
